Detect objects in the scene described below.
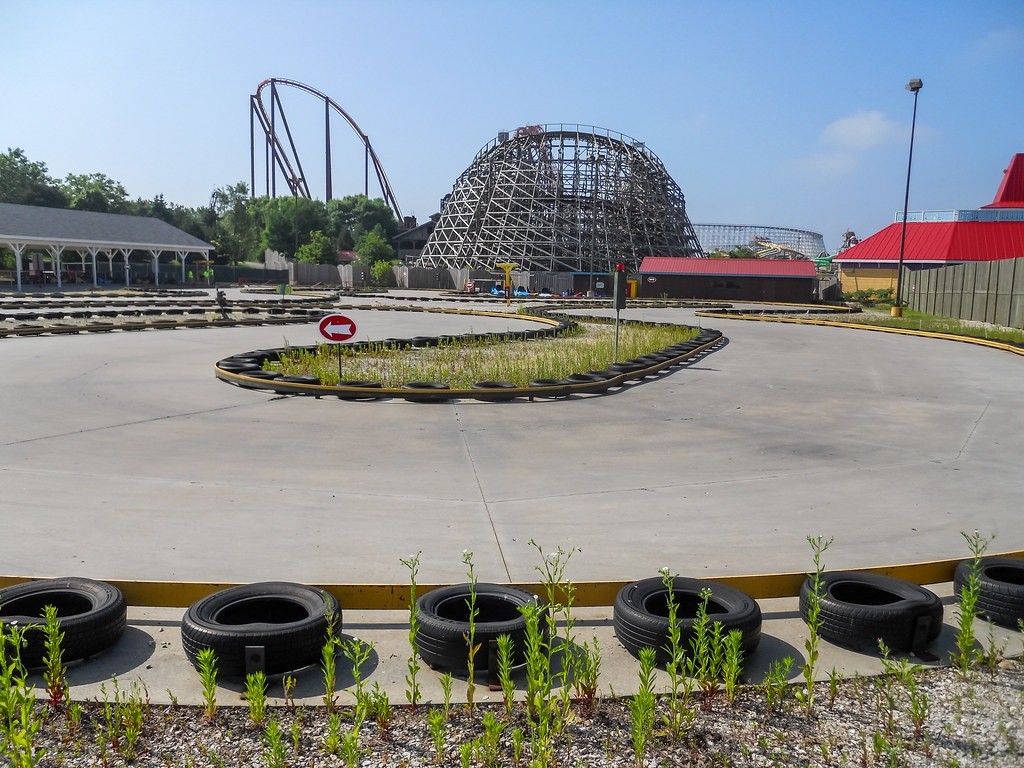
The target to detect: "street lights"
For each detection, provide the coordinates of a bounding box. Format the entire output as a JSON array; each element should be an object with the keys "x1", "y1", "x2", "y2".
[
  {"x1": 798, "y1": 237, "x2": 800, "y2": 253},
  {"x1": 287, "y1": 178, "x2": 304, "y2": 286},
  {"x1": 890, "y1": 78, "x2": 924, "y2": 317}
]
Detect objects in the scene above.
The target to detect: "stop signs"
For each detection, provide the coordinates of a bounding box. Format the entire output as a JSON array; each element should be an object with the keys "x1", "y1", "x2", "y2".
[{"x1": 647, "y1": 277, "x2": 657, "y2": 284}]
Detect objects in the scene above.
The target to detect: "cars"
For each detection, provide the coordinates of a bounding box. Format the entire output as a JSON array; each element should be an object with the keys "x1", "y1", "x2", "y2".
[{"x1": 491, "y1": 286, "x2": 559, "y2": 296}]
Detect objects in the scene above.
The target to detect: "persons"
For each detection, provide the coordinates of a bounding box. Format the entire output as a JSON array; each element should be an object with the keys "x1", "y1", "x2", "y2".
[
  {"x1": 203, "y1": 267, "x2": 213, "y2": 281},
  {"x1": 188, "y1": 268, "x2": 193, "y2": 280}
]
